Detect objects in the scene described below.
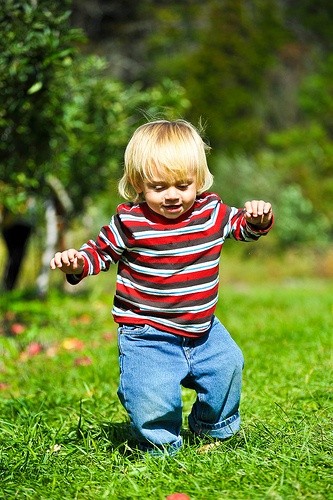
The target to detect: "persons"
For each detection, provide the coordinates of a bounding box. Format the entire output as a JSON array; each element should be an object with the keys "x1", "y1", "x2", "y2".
[{"x1": 50, "y1": 119, "x2": 274, "y2": 458}]
